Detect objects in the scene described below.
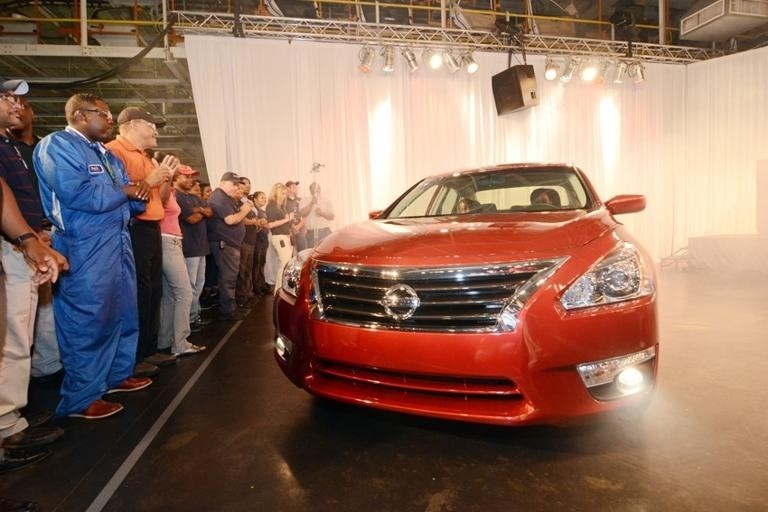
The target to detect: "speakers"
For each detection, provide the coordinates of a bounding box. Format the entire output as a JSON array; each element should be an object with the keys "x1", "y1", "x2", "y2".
[{"x1": 491, "y1": 64, "x2": 539, "y2": 117}]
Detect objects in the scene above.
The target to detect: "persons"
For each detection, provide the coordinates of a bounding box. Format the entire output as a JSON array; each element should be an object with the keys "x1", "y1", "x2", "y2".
[
  {"x1": 206, "y1": 171, "x2": 334, "y2": 319},
  {"x1": 0, "y1": 78, "x2": 212, "y2": 474},
  {"x1": 529, "y1": 189, "x2": 558, "y2": 207},
  {"x1": 457, "y1": 198, "x2": 477, "y2": 215}
]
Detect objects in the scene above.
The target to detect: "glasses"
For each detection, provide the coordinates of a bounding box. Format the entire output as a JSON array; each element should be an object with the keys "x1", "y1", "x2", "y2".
[
  {"x1": 72, "y1": 107, "x2": 113, "y2": 122},
  {"x1": 1, "y1": 92, "x2": 18, "y2": 104}
]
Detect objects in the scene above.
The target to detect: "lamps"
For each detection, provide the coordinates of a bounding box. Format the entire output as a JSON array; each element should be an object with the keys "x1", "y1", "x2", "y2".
[
  {"x1": 544, "y1": 50, "x2": 644, "y2": 84},
  {"x1": 358, "y1": 42, "x2": 479, "y2": 74}
]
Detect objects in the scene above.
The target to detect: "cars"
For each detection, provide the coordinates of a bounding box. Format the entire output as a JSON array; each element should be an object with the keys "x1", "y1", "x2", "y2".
[{"x1": 272, "y1": 160, "x2": 665, "y2": 432}]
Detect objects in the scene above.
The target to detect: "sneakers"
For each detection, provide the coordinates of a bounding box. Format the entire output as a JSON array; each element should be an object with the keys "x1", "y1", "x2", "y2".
[
  {"x1": 171, "y1": 344, "x2": 207, "y2": 355},
  {"x1": 190, "y1": 323, "x2": 201, "y2": 332},
  {"x1": 158, "y1": 336, "x2": 175, "y2": 350},
  {"x1": 198, "y1": 284, "x2": 220, "y2": 310},
  {"x1": 196, "y1": 316, "x2": 212, "y2": 325}
]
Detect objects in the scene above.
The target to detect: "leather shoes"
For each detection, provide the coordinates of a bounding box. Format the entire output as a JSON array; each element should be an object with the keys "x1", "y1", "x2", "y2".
[
  {"x1": 0, "y1": 496, "x2": 34, "y2": 512},
  {"x1": 105, "y1": 377, "x2": 153, "y2": 395},
  {"x1": 145, "y1": 352, "x2": 177, "y2": 366},
  {"x1": 67, "y1": 399, "x2": 124, "y2": 420},
  {"x1": 135, "y1": 362, "x2": 160, "y2": 375},
  {"x1": 219, "y1": 282, "x2": 273, "y2": 320},
  {"x1": 0, "y1": 404, "x2": 65, "y2": 473}
]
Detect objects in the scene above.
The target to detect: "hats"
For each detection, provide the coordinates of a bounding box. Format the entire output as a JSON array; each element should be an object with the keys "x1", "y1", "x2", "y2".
[
  {"x1": 0, "y1": 76, "x2": 24, "y2": 91},
  {"x1": 285, "y1": 181, "x2": 299, "y2": 187},
  {"x1": 222, "y1": 172, "x2": 245, "y2": 185},
  {"x1": 117, "y1": 107, "x2": 166, "y2": 128},
  {"x1": 177, "y1": 164, "x2": 200, "y2": 176}
]
